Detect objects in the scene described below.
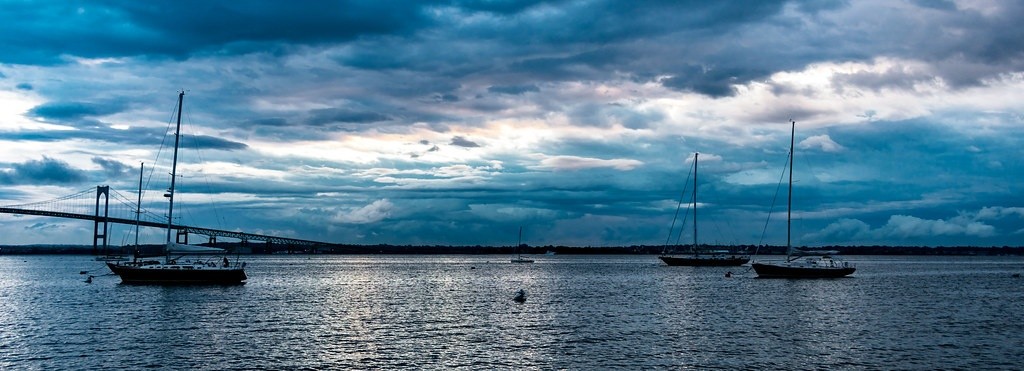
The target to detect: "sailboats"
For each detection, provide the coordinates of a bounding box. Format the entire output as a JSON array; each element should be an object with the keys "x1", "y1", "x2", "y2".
[
  {"x1": 106, "y1": 163, "x2": 162, "y2": 275},
  {"x1": 511, "y1": 226, "x2": 535, "y2": 263},
  {"x1": 659, "y1": 152, "x2": 751, "y2": 266},
  {"x1": 115, "y1": 89, "x2": 248, "y2": 283},
  {"x1": 751, "y1": 122, "x2": 857, "y2": 277}
]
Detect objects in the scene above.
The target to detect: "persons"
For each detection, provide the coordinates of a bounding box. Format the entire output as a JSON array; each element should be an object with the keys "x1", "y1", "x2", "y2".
[{"x1": 223, "y1": 258, "x2": 229, "y2": 267}]
